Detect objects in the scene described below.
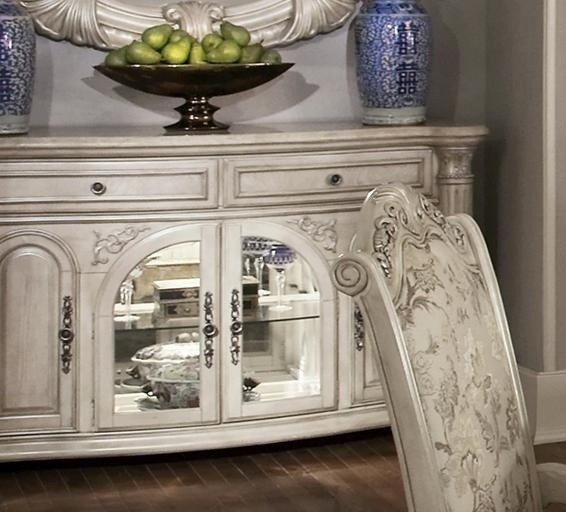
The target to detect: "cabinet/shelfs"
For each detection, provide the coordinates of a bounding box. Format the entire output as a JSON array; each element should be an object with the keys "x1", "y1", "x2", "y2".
[{"x1": 0, "y1": 122, "x2": 488, "y2": 465}]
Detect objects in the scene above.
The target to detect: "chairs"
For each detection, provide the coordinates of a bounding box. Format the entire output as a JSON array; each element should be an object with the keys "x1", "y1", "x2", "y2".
[{"x1": 328, "y1": 182, "x2": 566, "y2": 512}]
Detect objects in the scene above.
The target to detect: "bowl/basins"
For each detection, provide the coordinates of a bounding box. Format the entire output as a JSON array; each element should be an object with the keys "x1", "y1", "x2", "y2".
[{"x1": 129, "y1": 342, "x2": 200, "y2": 409}]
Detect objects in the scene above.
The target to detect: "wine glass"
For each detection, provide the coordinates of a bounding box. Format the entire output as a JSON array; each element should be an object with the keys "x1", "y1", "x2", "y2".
[
  {"x1": 113, "y1": 264, "x2": 142, "y2": 322},
  {"x1": 242, "y1": 235, "x2": 298, "y2": 312}
]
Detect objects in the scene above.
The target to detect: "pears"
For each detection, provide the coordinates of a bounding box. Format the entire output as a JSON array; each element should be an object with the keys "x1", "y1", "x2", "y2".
[{"x1": 105, "y1": 21, "x2": 282, "y2": 67}]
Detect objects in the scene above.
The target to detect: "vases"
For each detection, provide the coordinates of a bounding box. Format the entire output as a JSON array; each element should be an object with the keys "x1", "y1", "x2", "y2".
[
  {"x1": 353, "y1": 1, "x2": 433, "y2": 126},
  {"x1": 0, "y1": 0, "x2": 36, "y2": 136}
]
